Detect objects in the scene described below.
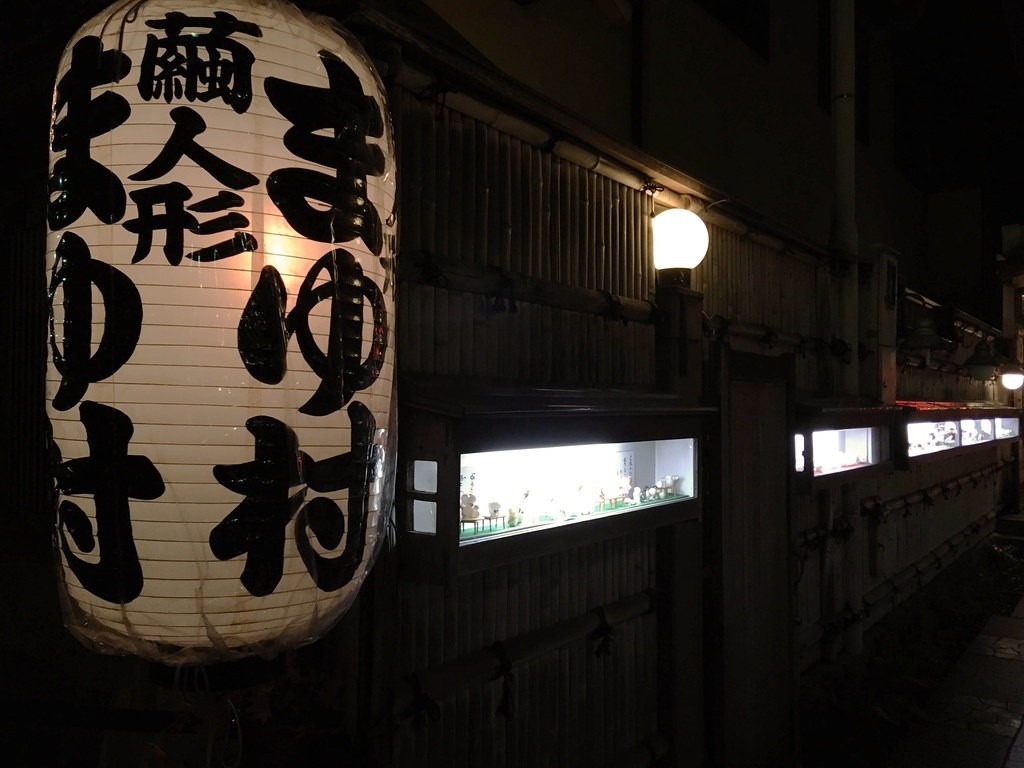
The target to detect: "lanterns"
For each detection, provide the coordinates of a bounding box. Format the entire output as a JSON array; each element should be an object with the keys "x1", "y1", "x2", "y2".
[{"x1": 45, "y1": 0, "x2": 398, "y2": 692}]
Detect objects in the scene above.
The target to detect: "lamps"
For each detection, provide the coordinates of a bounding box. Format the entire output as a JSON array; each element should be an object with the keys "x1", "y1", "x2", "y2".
[
  {"x1": 999, "y1": 338, "x2": 1024, "y2": 391},
  {"x1": 965, "y1": 330, "x2": 999, "y2": 383},
  {"x1": 653, "y1": 207, "x2": 709, "y2": 287}
]
